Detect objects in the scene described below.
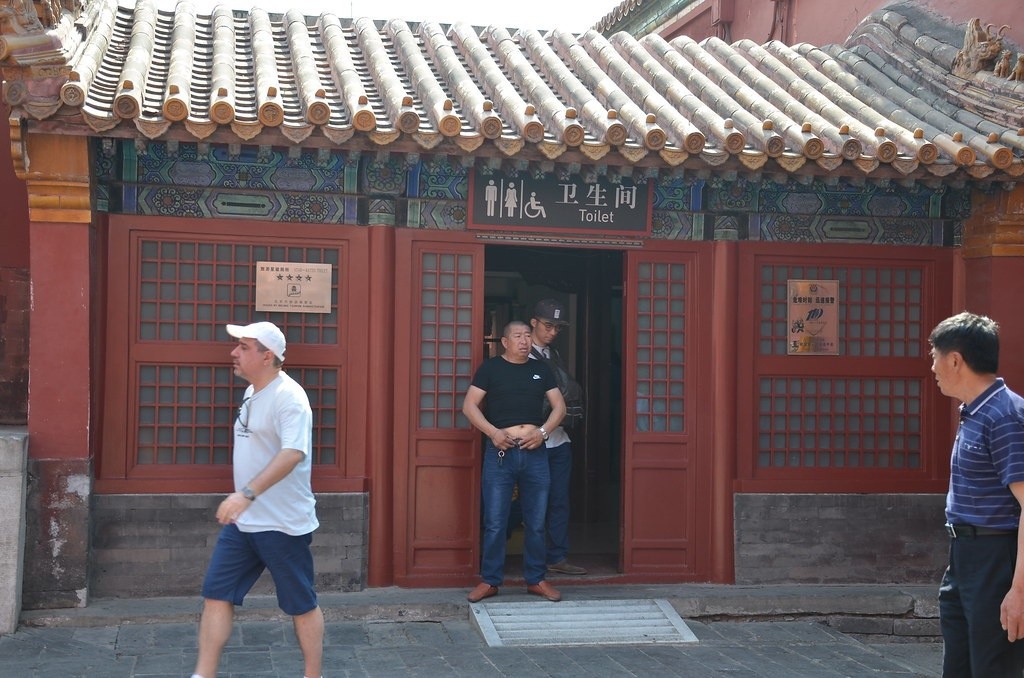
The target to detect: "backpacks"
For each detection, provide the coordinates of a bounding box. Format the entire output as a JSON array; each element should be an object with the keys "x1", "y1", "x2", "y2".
[{"x1": 531, "y1": 342, "x2": 585, "y2": 426}]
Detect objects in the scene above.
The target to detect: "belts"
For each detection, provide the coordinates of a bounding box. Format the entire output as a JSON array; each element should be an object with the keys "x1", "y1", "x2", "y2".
[
  {"x1": 510, "y1": 439, "x2": 525, "y2": 446},
  {"x1": 945, "y1": 521, "x2": 1019, "y2": 538}
]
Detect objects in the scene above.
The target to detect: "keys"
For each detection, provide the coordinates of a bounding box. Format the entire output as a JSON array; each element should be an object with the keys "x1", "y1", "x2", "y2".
[{"x1": 498, "y1": 449, "x2": 505, "y2": 467}]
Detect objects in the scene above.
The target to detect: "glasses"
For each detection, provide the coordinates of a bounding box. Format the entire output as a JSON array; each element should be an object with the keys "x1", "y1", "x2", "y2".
[
  {"x1": 235, "y1": 397, "x2": 249, "y2": 428},
  {"x1": 532, "y1": 316, "x2": 564, "y2": 333}
]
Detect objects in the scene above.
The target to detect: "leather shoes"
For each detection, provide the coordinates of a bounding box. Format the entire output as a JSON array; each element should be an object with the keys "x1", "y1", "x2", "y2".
[{"x1": 526, "y1": 580, "x2": 561, "y2": 601}]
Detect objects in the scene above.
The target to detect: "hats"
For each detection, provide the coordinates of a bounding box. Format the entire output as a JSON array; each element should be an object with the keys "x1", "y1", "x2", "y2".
[
  {"x1": 532, "y1": 298, "x2": 571, "y2": 329},
  {"x1": 225, "y1": 321, "x2": 286, "y2": 362}
]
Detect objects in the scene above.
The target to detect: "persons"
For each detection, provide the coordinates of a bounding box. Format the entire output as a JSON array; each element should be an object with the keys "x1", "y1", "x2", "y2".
[
  {"x1": 191, "y1": 321, "x2": 324, "y2": 678},
  {"x1": 506, "y1": 299, "x2": 587, "y2": 574},
  {"x1": 929, "y1": 312, "x2": 1024, "y2": 678},
  {"x1": 462, "y1": 322, "x2": 567, "y2": 603}
]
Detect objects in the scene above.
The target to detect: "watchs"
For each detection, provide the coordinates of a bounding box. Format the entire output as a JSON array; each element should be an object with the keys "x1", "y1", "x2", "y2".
[
  {"x1": 241, "y1": 487, "x2": 256, "y2": 501},
  {"x1": 539, "y1": 427, "x2": 549, "y2": 441}
]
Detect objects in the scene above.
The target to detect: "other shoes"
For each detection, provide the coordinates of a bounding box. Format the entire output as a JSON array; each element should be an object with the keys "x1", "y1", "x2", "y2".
[
  {"x1": 467, "y1": 582, "x2": 498, "y2": 603},
  {"x1": 546, "y1": 558, "x2": 591, "y2": 575}
]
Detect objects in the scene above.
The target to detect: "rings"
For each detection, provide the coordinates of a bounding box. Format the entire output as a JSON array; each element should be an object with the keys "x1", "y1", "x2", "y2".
[{"x1": 230, "y1": 514, "x2": 237, "y2": 520}]
